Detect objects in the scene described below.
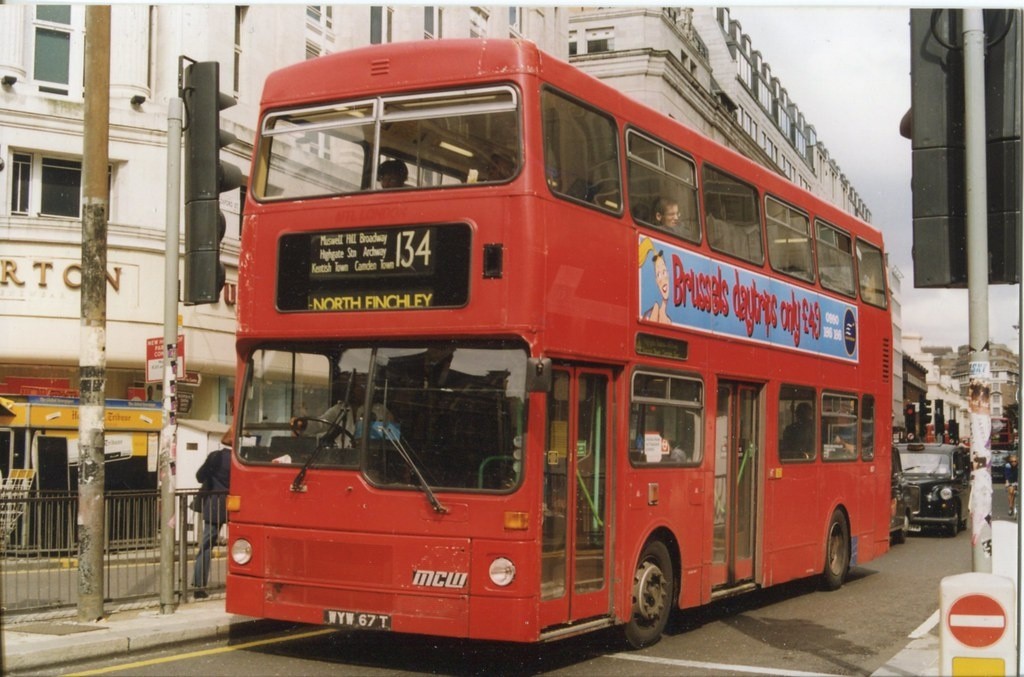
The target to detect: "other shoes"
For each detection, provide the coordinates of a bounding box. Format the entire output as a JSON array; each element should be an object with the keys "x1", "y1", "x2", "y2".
[{"x1": 193, "y1": 590, "x2": 208, "y2": 600}]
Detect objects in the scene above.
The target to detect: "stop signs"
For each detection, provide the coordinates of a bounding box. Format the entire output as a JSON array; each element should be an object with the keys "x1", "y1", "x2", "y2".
[{"x1": 945, "y1": 594, "x2": 1009, "y2": 650}]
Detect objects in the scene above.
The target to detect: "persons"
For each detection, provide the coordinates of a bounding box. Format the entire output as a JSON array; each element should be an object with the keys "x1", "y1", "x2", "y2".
[
  {"x1": 653, "y1": 196, "x2": 681, "y2": 232},
  {"x1": 190, "y1": 427, "x2": 234, "y2": 600},
  {"x1": 980, "y1": 382, "x2": 991, "y2": 409},
  {"x1": 147, "y1": 386, "x2": 153, "y2": 401},
  {"x1": 822, "y1": 409, "x2": 854, "y2": 455},
  {"x1": 492, "y1": 153, "x2": 515, "y2": 179},
  {"x1": 1004, "y1": 454, "x2": 1019, "y2": 515},
  {"x1": 290, "y1": 368, "x2": 394, "y2": 450},
  {"x1": 377, "y1": 159, "x2": 410, "y2": 188},
  {"x1": 782, "y1": 403, "x2": 815, "y2": 454},
  {"x1": 970, "y1": 379, "x2": 983, "y2": 408}
]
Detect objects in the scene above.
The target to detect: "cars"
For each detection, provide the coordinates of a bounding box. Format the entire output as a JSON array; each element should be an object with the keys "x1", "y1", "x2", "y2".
[
  {"x1": 894, "y1": 442, "x2": 975, "y2": 538},
  {"x1": 884, "y1": 443, "x2": 919, "y2": 545},
  {"x1": 993, "y1": 450, "x2": 1011, "y2": 483}
]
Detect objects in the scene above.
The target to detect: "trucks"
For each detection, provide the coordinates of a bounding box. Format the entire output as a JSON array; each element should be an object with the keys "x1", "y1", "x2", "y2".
[{"x1": 991, "y1": 416, "x2": 1015, "y2": 450}]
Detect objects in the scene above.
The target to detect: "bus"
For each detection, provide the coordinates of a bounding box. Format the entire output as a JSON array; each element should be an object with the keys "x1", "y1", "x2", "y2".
[
  {"x1": 1, "y1": 396, "x2": 160, "y2": 559},
  {"x1": 217, "y1": 33, "x2": 896, "y2": 652}
]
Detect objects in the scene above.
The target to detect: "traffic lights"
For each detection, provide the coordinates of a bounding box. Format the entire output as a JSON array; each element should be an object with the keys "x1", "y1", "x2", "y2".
[
  {"x1": 919, "y1": 396, "x2": 932, "y2": 433},
  {"x1": 906, "y1": 404, "x2": 918, "y2": 438},
  {"x1": 949, "y1": 419, "x2": 957, "y2": 438},
  {"x1": 895, "y1": 10, "x2": 955, "y2": 288},
  {"x1": 174, "y1": 53, "x2": 244, "y2": 313}
]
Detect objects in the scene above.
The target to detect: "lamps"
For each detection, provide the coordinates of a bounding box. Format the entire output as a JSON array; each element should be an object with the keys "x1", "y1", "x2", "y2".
[
  {"x1": 131, "y1": 96, "x2": 145, "y2": 104},
  {"x1": 2, "y1": 75, "x2": 17, "y2": 86}
]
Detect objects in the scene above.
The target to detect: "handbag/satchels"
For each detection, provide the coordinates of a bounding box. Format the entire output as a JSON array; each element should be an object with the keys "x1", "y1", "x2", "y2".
[{"x1": 188, "y1": 475, "x2": 213, "y2": 513}]
